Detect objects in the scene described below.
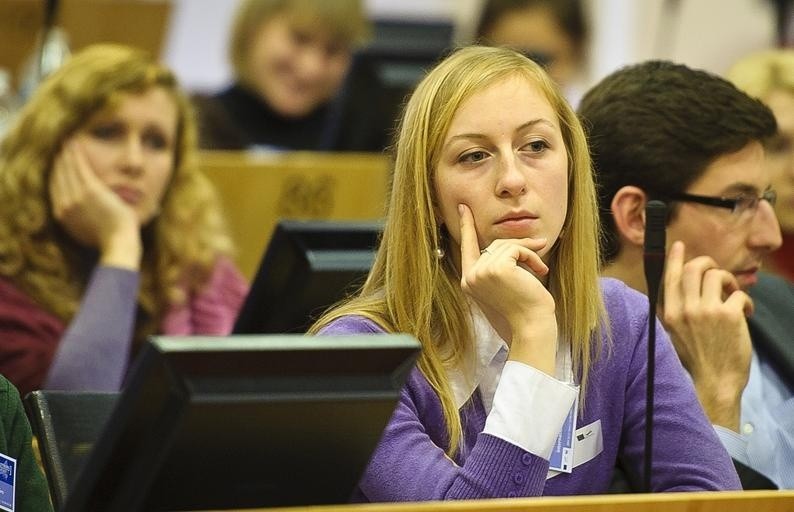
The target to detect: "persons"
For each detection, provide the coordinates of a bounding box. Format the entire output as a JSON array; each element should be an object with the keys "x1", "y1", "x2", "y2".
[
  {"x1": 473, "y1": 0, "x2": 591, "y2": 88},
  {"x1": 574, "y1": 59, "x2": 794, "y2": 491},
  {"x1": 0, "y1": 42, "x2": 252, "y2": 395},
  {"x1": 310, "y1": 42, "x2": 748, "y2": 503},
  {"x1": 194, "y1": 2, "x2": 393, "y2": 149},
  {"x1": 0, "y1": 373, "x2": 55, "y2": 512}
]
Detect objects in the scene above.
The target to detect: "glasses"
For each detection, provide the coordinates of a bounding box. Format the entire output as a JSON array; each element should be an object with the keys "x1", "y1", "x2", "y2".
[{"x1": 673, "y1": 189, "x2": 777, "y2": 225}]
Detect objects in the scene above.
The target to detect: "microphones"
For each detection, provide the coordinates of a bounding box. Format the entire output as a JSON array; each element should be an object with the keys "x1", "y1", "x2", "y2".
[{"x1": 640, "y1": 198, "x2": 670, "y2": 490}]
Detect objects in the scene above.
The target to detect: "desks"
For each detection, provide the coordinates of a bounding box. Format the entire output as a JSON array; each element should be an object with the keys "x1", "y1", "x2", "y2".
[{"x1": 198, "y1": 142, "x2": 391, "y2": 288}]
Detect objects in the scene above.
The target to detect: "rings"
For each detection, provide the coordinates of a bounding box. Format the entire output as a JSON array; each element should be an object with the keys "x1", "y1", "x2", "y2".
[{"x1": 481, "y1": 248, "x2": 491, "y2": 255}]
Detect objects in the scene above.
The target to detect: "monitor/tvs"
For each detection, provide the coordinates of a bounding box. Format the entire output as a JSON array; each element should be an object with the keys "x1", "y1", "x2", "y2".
[
  {"x1": 59, "y1": 329, "x2": 424, "y2": 511},
  {"x1": 227, "y1": 216, "x2": 386, "y2": 334}
]
